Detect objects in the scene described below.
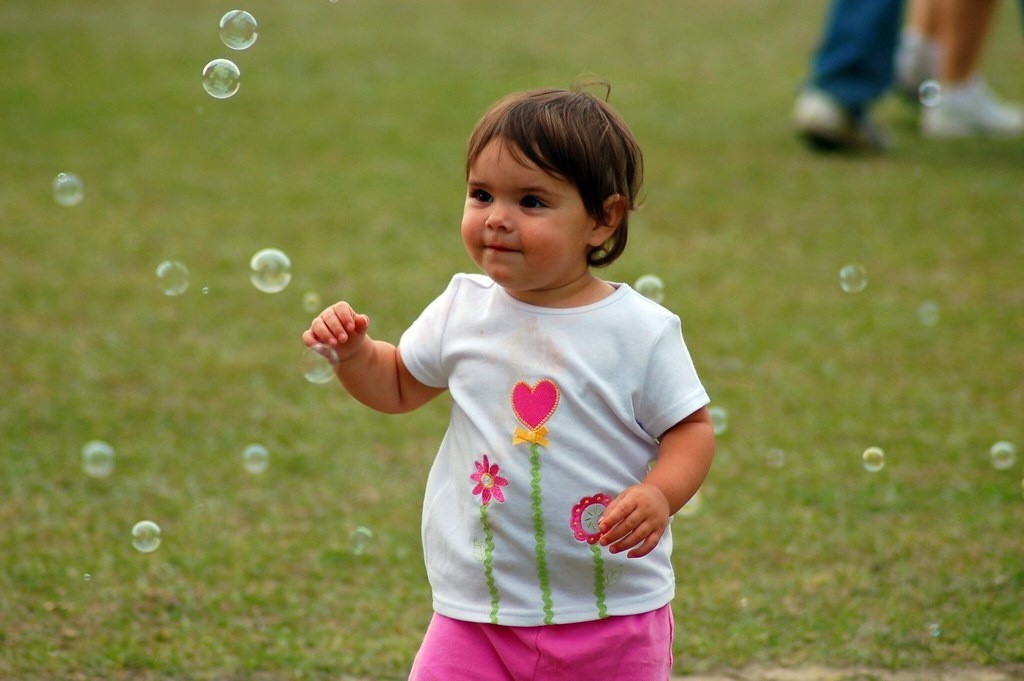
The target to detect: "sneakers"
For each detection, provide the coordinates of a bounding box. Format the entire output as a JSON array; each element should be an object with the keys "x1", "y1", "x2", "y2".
[
  {"x1": 788, "y1": 90, "x2": 887, "y2": 154},
  {"x1": 896, "y1": 35, "x2": 943, "y2": 94},
  {"x1": 920, "y1": 77, "x2": 1023, "y2": 139}
]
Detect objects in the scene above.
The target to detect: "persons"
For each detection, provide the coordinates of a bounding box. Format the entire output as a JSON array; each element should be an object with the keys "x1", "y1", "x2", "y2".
[
  {"x1": 790, "y1": 0, "x2": 1024, "y2": 154},
  {"x1": 302, "y1": 87, "x2": 715, "y2": 681}
]
